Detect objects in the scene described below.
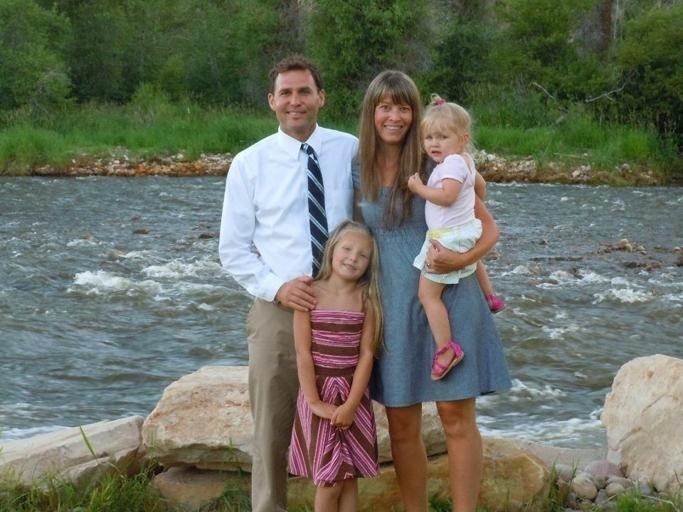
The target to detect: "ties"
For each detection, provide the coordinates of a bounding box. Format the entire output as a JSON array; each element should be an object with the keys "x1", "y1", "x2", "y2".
[{"x1": 301, "y1": 143, "x2": 330, "y2": 280}]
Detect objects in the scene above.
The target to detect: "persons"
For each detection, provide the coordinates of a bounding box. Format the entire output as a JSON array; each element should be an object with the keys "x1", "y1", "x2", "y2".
[
  {"x1": 286, "y1": 219, "x2": 383, "y2": 511},
  {"x1": 218, "y1": 54, "x2": 359, "y2": 512},
  {"x1": 405, "y1": 93, "x2": 506, "y2": 381},
  {"x1": 352, "y1": 71, "x2": 513, "y2": 511}
]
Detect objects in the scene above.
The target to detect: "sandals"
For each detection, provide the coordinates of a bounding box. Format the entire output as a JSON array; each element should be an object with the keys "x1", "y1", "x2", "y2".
[
  {"x1": 431, "y1": 344, "x2": 464, "y2": 381},
  {"x1": 485, "y1": 294, "x2": 505, "y2": 314}
]
